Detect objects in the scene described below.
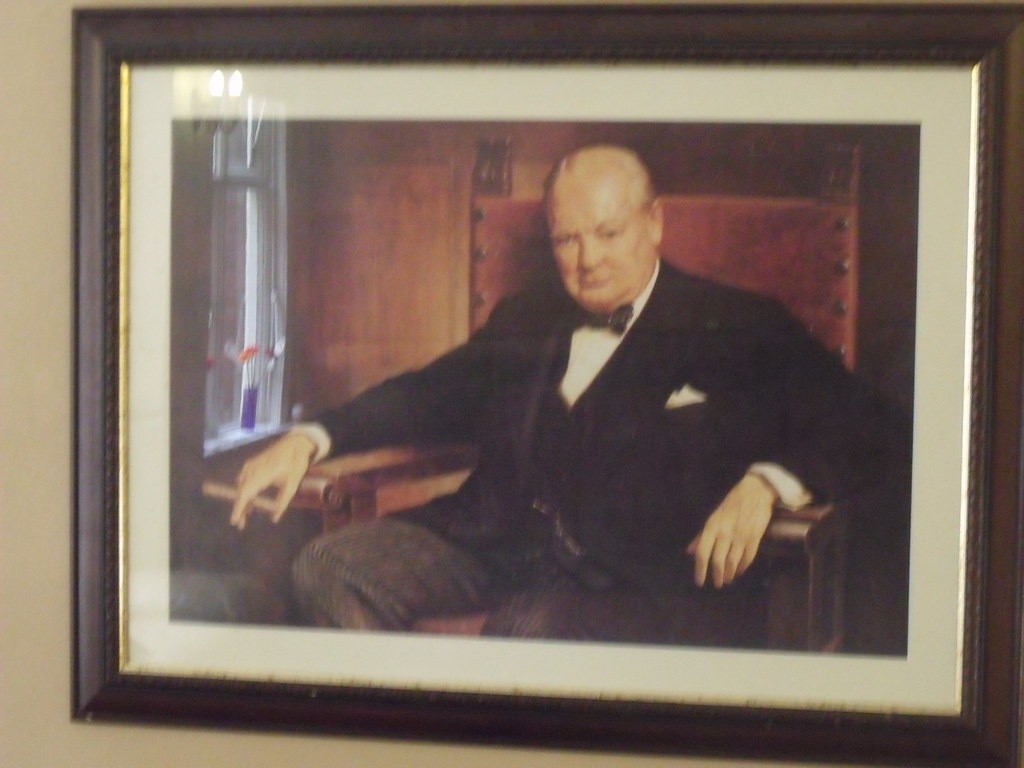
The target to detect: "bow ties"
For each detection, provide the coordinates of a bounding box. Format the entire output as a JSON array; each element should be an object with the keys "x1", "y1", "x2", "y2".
[{"x1": 565, "y1": 305, "x2": 632, "y2": 333}]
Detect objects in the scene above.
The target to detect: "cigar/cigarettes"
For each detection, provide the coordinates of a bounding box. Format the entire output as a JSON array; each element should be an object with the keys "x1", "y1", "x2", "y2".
[{"x1": 200, "y1": 478, "x2": 277, "y2": 513}]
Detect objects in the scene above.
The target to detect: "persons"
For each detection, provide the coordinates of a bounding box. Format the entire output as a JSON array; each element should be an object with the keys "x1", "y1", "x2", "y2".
[{"x1": 229, "y1": 145, "x2": 881, "y2": 645}]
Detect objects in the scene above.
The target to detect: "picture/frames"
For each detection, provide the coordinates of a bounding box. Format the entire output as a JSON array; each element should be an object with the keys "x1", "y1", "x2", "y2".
[{"x1": 72, "y1": 0, "x2": 1024, "y2": 768}]
